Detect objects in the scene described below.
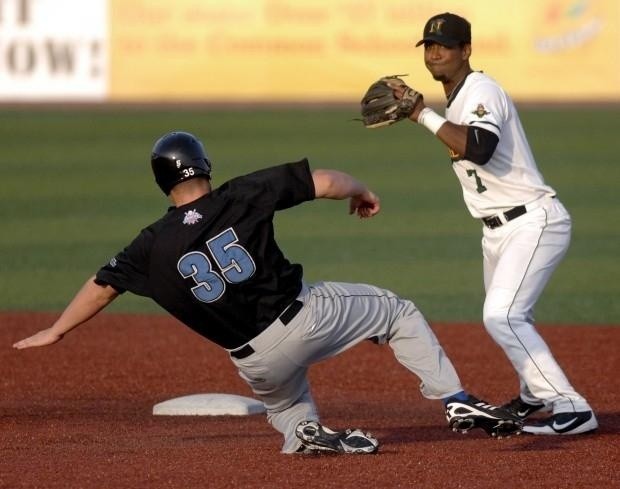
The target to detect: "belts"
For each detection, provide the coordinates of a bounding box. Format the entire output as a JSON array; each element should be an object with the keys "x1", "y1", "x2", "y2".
[
  {"x1": 481, "y1": 191, "x2": 556, "y2": 230},
  {"x1": 229, "y1": 298, "x2": 305, "y2": 360}
]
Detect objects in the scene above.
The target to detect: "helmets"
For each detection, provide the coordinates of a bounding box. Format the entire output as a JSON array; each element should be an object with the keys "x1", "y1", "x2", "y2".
[{"x1": 150, "y1": 131, "x2": 213, "y2": 197}]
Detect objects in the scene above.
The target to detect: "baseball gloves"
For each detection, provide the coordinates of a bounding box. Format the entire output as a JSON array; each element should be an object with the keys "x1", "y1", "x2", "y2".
[{"x1": 353, "y1": 74, "x2": 423, "y2": 130}]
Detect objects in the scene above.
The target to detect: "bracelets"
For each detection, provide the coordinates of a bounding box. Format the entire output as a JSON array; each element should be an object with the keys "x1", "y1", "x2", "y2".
[{"x1": 418, "y1": 107, "x2": 448, "y2": 135}]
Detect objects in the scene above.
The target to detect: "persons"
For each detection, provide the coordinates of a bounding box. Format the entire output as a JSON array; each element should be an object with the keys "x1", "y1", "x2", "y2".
[
  {"x1": 386, "y1": 13, "x2": 599, "y2": 435},
  {"x1": 13, "y1": 132, "x2": 524, "y2": 454}
]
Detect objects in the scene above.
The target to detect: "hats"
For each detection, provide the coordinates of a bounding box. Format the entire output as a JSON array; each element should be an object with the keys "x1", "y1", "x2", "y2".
[{"x1": 414, "y1": 12, "x2": 471, "y2": 49}]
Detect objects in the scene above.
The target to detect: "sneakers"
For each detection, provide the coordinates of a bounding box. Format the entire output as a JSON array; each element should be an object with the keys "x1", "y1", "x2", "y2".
[
  {"x1": 443, "y1": 393, "x2": 525, "y2": 440},
  {"x1": 496, "y1": 394, "x2": 555, "y2": 419},
  {"x1": 520, "y1": 407, "x2": 600, "y2": 436},
  {"x1": 294, "y1": 419, "x2": 380, "y2": 456}
]
